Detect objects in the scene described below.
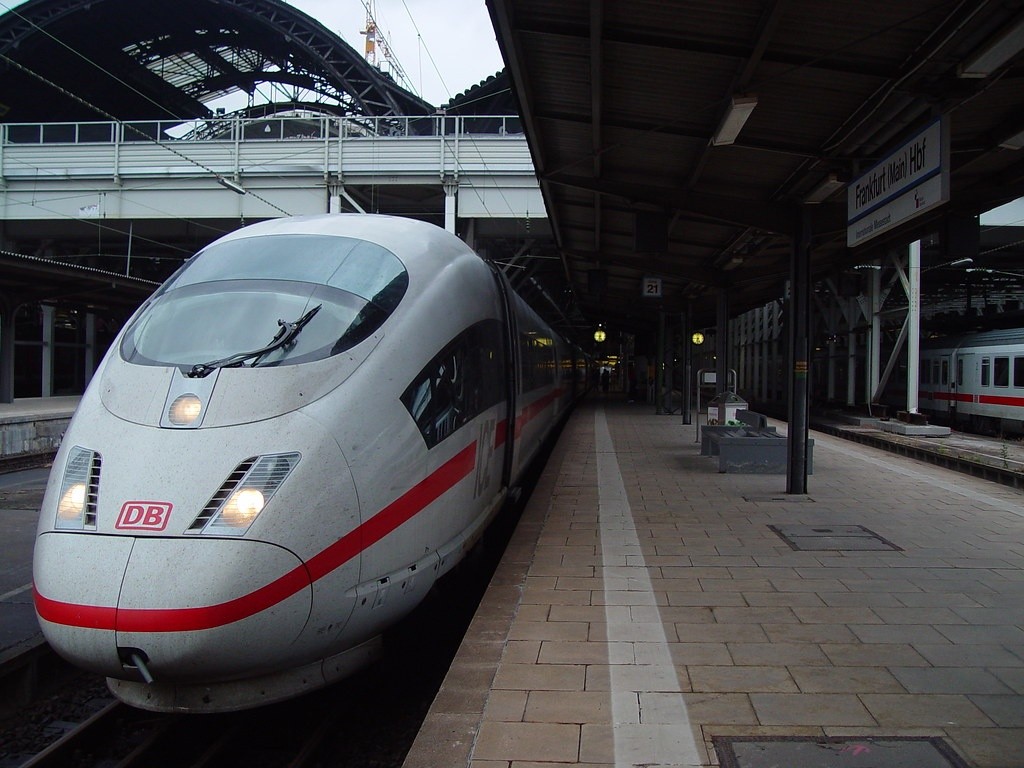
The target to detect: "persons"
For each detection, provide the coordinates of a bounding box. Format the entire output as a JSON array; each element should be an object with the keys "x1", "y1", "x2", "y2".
[{"x1": 601, "y1": 369, "x2": 617, "y2": 393}]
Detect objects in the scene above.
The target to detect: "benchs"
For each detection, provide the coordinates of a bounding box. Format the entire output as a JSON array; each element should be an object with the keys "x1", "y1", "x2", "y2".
[{"x1": 701, "y1": 409, "x2": 814, "y2": 475}]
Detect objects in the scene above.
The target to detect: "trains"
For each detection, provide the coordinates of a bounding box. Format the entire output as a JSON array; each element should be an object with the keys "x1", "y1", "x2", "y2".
[
  {"x1": 814, "y1": 326, "x2": 1024, "y2": 435},
  {"x1": 30, "y1": 211, "x2": 598, "y2": 717}
]
